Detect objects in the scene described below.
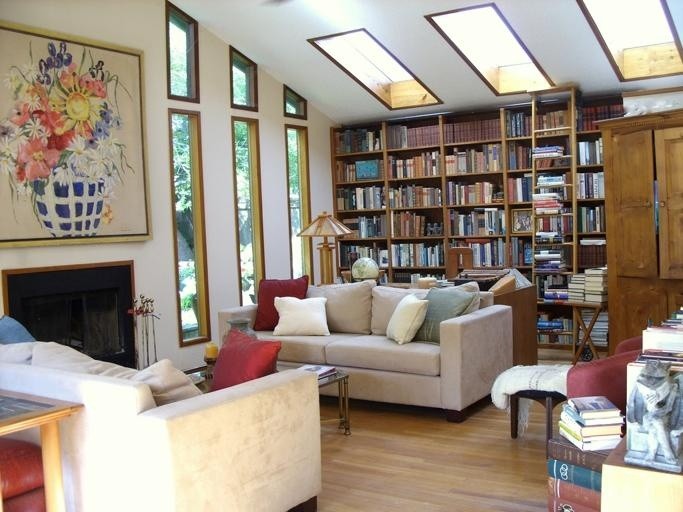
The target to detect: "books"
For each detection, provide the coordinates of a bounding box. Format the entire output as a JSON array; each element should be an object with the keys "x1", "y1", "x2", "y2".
[
  {"x1": 536, "y1": 172, "x2": 573, "y2": 202},
  {"x1": 507, "y1": 173, "x2": 532, "y2": 202},
  {"x1": 536, "y1": 311, "x2": 573, "y2": 346},
  {"x1": 389, "y1": 184, "x2": 442, "y2": 208},
  {"x1": 576, "y1": 172, "x2": 605, "y2": 199},
  {"x1": 509, "y1": 237, "x2": 533, "y2": 266},
  {"x1": 547, "y1": 396, "x2": 627, "y2": 512},
  {"x1": 569, "y1": 267, "x2": 609, "y2": 303},
  {"x1": 506, "y1": 109, "x2": 532, "y2": 137},
  {"x1": 388, "y1": 151, "x2": 441, "y2": 179},
  {"x1": 578, "y1": 138, "x2": 604, "y2": 166},
  {"x1": 534, "y1": 231, "x2": 574, "y2": 274},
  {"x1": 446, "y1": 208, "x2": 506, "y2": 236},
  {"x1": 578, "y1": 238, "x2": 607, "y2": 267},
  {"x1": 387, "y1": 125, "x2": 439, "y2": 149},
  {"x1": 446, "y1": 181, "x2": 504, "y2": 205},
  {"x1": 538, "y1": 144, "x2": 572, "y2": 167},
  {"x1": 337, "y1": 186, "x2": 386, "y2": 210},
  {"x1": 446, "y1": 144, "x2": 503, "y2": 174},
  {"x1": 334, "y1": 127, "x2": 380, "y2": 153},
  {"x1": 338, "y1": 241, "x2": 389, "y2": 268},
  {"x1": 394, "y1": 272, "x2": 445, "y2": 283},
  {"x1": 577, "y1": 105, "x2": 625, "y2": 131},
  {"x1": 577, "y1": 206, "x2": 606, "y2": 233},
  {"x1": 579, "y1": 309, "x2": 609, "y2": 347},
  {"x1": 343, "y1": 214, "x2": 386, "y2": 239},
  {"x1": 336, "y1": 160, "x2": 384, "y2": 182},
  {"x1": 342, "y1": 271, "x2": 388, "y2": 285},
  {"x1": 533, "y1": 193, "x2": 563, "y2": 214},
  {"x1": 449, "y1": 238, "x2": 506, "y2": 267},
  {"x1": 509, "y1": 140, "x2": 532, "y2": 170},
  {"x1": 391, "y1": 209, "x2": 425, "y2": 236},
  {"x1": 539, "y1": 110, "x2": 570, "y2": 134},
  {"x1": 443, "y1": 119, "x2": 501, "y2": 144},
  {"x1": 535, "y1": 274, "x2": 572, "y2": 305},
  {"x1": 392, "y1": 242, "x2": 443, "y2": 267},
  {"x1": 535, "y1": 208, "x2": 573, "y2": 234},
  {"x1": 298, "y1": 365, "x2": 336, "y2": 379}
]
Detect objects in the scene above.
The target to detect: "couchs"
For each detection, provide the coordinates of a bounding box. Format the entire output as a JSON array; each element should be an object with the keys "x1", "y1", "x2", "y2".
[
  {"x1": 217, "y1": 289, "x2": 513, "y2": 424},
  {"x1": 1, "y1": 363, "x2": 321, "y2": 512}
]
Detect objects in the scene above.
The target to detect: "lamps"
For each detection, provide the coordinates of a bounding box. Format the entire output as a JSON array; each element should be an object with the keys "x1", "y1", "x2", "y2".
[{"x1": 297, "y1": 213, "x2": 353, "y2": 286}]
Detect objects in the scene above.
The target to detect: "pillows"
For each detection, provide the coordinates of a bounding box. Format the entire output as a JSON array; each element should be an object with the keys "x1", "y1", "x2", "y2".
[
  {"x1": 211, "y1": 329, "x2": 281, "y2": 393},
  {"x1": 182, "y1": 362, "x2": 352, "y2": 436},
  {"x1": 371, "y1": 285, "x2": 433, "y2": 337},
  {"x1": 254, "y1": 276, "x2": 310, "y2": 331},
  {"x1": 448, "y1": 282, "x2": 480, "y2": 315},
  {"x1": 386, "y1": 294, "x2": 429, "y2": 344},
  {"x1": 416, "y1": 287, "x2": 475, "y2": 345},
  {"x1": 35, "y1": 342, "x2": 203, "y2": 403},
  {"x1": 273, "y1": 297, "x2": 330, "y2": 337},
  {"x1": 306, "y1": 280, "x2": 376, "y2": 335}
]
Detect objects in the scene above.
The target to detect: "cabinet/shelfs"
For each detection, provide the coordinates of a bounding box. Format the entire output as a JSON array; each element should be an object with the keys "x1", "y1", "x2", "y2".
[
  {"x1": 607, "y1": 276, "x2": 682, "y2": 357},
  {"x1": 592, "y1": 107, "x2": 683, "y2": 280},
  {"x1": 329, "y1": 91, "x2": 625, "y2": 352}
]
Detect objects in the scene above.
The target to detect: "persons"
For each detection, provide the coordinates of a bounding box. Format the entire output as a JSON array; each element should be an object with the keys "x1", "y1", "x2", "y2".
[{"x1": 623, "y1": 307, "x2": 682, "y2": 473}]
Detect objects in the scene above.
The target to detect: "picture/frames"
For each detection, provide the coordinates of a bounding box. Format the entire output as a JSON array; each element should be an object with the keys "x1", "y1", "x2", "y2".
[{"x1": 1, "y1": 20, "x2": 153, "y2": 249}]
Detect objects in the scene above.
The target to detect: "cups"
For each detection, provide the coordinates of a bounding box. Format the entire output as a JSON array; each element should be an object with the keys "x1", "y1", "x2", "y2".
[{"x1": 410, "y1": 274, "x2": 422, "y2": 287}]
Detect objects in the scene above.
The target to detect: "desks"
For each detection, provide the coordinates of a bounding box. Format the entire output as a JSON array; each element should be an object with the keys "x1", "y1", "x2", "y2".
[{"x1": 2, "y1": 389, "x2": 84, "y2": 511}]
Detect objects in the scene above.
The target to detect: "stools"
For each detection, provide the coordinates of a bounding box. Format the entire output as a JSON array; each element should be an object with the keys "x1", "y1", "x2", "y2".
[{"x1": 508, "y1": 389, "x2": 567, "y2": 461}]
